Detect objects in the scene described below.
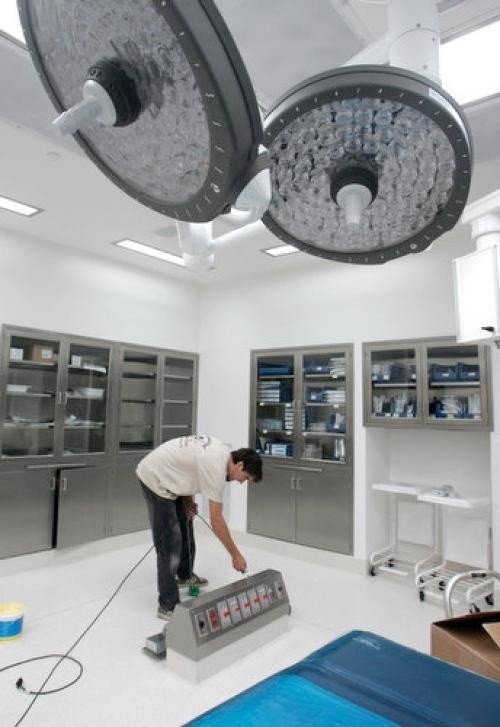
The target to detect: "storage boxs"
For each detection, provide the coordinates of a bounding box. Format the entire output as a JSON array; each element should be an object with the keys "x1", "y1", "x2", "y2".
[
  {"x1": 25, "y1": 343, "x2": 54, "y2": 362},
  {"x1": 430, "y1": 609, "x2": 500, "y2": 684}
]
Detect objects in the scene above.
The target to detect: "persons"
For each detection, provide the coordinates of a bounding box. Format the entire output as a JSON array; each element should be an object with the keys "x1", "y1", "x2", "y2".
[{"x1": 134, "y1": 432, "x2": 263, "y2": 619}]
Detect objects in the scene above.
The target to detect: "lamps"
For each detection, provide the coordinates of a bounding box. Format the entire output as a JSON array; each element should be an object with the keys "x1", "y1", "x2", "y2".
[
  {"x1": 261, "y1": 63, "x2": 474, "y2": 266},
  {"x1": 16, "y1": 1, "x2": 273, "y2": 241}
]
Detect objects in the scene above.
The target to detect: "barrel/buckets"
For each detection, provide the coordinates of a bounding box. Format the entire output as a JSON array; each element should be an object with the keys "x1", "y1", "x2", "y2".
[{"x1": 0, "y1": 603, "x2": 24, "y2": 641}]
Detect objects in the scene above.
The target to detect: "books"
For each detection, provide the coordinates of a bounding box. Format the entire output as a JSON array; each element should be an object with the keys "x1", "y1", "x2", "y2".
[
  {"x1": 373, "y1": 359, "x2": 483, "y2": 420},
  {"x1": 257, "y1": 353, "x2": 348, "y2": 460}
]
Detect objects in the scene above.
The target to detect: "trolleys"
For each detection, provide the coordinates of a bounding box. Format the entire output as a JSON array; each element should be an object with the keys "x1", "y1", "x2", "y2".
[{"x1": 367, "y1": 480, "x2": 500, "y2": 621}]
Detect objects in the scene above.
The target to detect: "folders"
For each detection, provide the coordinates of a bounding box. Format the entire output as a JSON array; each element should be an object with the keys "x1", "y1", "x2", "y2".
[
  {"x1": 257, "y1": 364, "x2": 292, "y2": 403},
  {"x1": 266, "y1": 439, "x2": 293, "y2": 456},
  {"x1": 303, "y1": 365, "x2": 332, "y2": 373},
  {"x1": 306, "y1": 387, "x2": 337, "y2": 403}
]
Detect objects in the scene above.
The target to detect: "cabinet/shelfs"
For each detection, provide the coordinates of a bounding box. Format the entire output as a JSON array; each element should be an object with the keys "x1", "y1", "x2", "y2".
[
  {"x1": 0, "y1": 322, "x2": 200, "y2": 560},
  {"x1": 247, "y1": 343, "x2": 354, "y2": 558},
  {"x1": 362, "y1": 335, "x2": 493, "y2": 431}
]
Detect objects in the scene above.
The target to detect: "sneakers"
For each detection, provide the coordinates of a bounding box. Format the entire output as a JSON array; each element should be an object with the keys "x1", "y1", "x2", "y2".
[
  {"x1": 175, "y1": 571, "x2": 209, "y2": 589},
  {"x1": 156, "y1": 604, "x2": 174, "y2": 622}
]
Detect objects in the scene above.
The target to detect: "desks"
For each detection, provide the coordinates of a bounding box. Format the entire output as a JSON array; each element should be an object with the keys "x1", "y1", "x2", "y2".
[{"x1": 369, "y1": 482, "x2": 492, "y2": 613}]
